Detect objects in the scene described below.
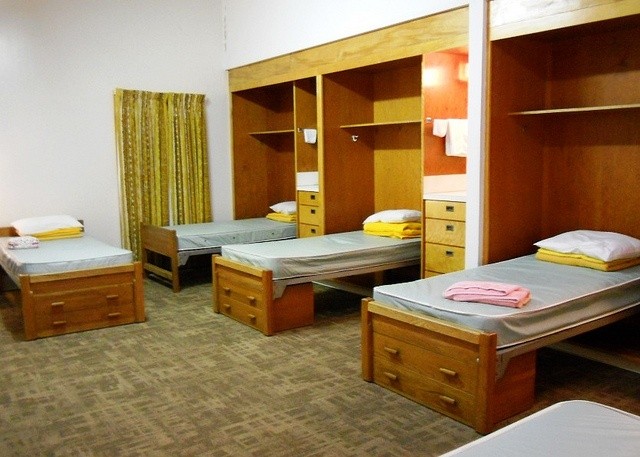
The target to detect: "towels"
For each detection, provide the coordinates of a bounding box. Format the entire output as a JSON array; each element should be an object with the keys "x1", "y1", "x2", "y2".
[
  {"x1": 449, "y1": 280, "x2": 533, "y2": 308},
  {"x1": 304, "y1": 128, "x2": 317, "y2": 143},
  {"x1": 433, "y1": 118, "x2": 448, "y2": 136},
  {"x1": 445, "y1": 118, "x2": 467, "y2": 156}
]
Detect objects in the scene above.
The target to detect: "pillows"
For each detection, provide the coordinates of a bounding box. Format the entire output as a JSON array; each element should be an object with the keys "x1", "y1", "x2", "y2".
[
  {"x1": 534, "y1": 229, "x2": 639, "y2": 270},
  {"x1": 365, "y1": 210, "x2": 441, "y2": 240},
  {"x1": 12, "y1": 216, "x2": 83, "y2": 239},
  {"x1": 267, "y1": 203, "x2": 298, "y2": 224}
]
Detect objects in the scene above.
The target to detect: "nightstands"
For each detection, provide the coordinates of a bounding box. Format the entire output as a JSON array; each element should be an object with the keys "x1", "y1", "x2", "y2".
[
  {"x1": 296, "y1": 189, "x2": 322, "y2": 239},
  {"x1": 423, "y1": 200, "x2": 466, "y2": 274}
]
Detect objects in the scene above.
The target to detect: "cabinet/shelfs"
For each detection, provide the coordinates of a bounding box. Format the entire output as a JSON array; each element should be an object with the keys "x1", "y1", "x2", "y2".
[
  {"x1": 328, "y1": 22, "x2": 427, "y2": 233},
  {"x1": 225, "y1": 47, "x2": 324, "y2": 235},
  {"x1": 489, "y1": 1, "x2": 639, "y2": 259}
]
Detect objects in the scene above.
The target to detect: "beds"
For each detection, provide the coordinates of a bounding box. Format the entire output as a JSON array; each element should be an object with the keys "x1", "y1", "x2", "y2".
[
  {"x1": 4, "y1": 219, "x2": 147, "y2": 344},
  {"x1": 361, "y1": 250, "x2": 639, "y2": 435},
  {"x1": 211, "y1": 229, "x2": 421, "y2": 338},
  {"x1": 143, "y1": 209, "x2": 296, "y2": 290}
]
